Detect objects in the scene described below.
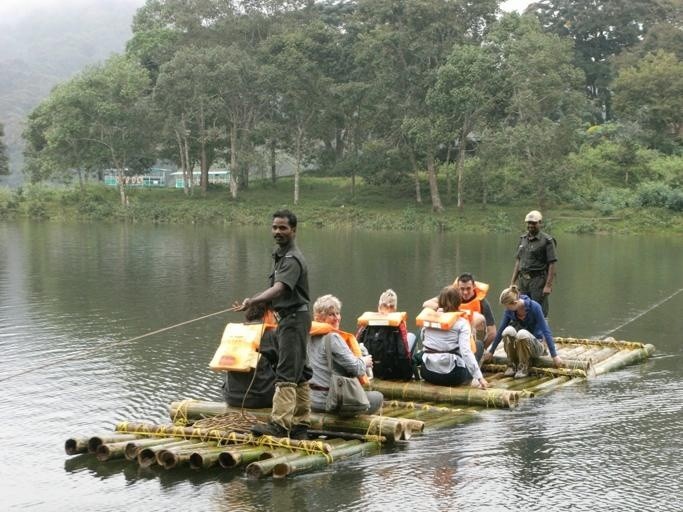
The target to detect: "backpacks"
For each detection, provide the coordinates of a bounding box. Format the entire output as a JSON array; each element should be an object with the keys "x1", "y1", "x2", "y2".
[{"x1": 360, "y1": 326, "x2": 407, "y2": 381}]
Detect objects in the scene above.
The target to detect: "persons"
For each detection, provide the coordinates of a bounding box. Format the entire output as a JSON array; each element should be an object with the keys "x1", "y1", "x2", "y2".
[
  {"x1": 229, "y1": 209, "x2": 313, "y2": 443},
  {"x1": 209, "y1": 293, "x2": 281, "y2": 410},
  {"x1": 353, "y1": 287, "x2": 418, "y2": 381},
  {"x1": 415, "y1": 284, "x2": 489, "y2": 390},
  {"x1": 510, "y1": 211, "x2": 558, "y2": 356},
  {"x1": 481, "y1": 285, "x2": 563, "y2": 381},
  {"x1": 422, "y1": 272, "x2": 496, "y2": 369},
  {"x1": 306, "y1": 292, "x2": 385, "y2": 417}
]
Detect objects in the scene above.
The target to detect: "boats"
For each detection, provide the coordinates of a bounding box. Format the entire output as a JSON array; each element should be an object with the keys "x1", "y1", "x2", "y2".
[{"x1": 58, "y1": 334, "x2": 659, "y2": 488}]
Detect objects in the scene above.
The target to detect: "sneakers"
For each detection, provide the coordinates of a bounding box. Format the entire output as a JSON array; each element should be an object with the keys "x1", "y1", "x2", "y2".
[
  {"x1": 251, "y1": 421, "x2": 309, "y2": 440},
  {"x1": 515, "y1": 371, "x2": 528, "y2": 378},
  {"x1": 504, "y1": 367, "x2": 516, "y2": 375}
]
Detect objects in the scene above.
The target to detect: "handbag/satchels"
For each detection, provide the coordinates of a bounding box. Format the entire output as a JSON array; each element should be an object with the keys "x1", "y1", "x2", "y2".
[{"x1": 325, "y1": 373, "x2": 370, "y2": 415}]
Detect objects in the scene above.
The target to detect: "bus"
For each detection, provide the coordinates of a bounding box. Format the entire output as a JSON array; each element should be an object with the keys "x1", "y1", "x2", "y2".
[
  {"x1": 169, "y1": 170, "x2": 230, "y2": 188},
  {"x1": 103, "y1": 165, "x2": 172, "y2": 187}
]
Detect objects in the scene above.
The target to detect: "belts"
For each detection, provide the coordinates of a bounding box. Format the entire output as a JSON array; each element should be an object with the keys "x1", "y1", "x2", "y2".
[
  {"x1": 273, "y1": 304, "x2": 308, "y2": 322},
  {"x1": 522, "y1": 272, "x2": 544, "y2": 279}
]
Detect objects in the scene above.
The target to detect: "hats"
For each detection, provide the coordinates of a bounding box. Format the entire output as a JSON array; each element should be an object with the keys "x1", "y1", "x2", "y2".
[{"x1": 525, "y1": 210, "x2": 542, "y2": 222}]
